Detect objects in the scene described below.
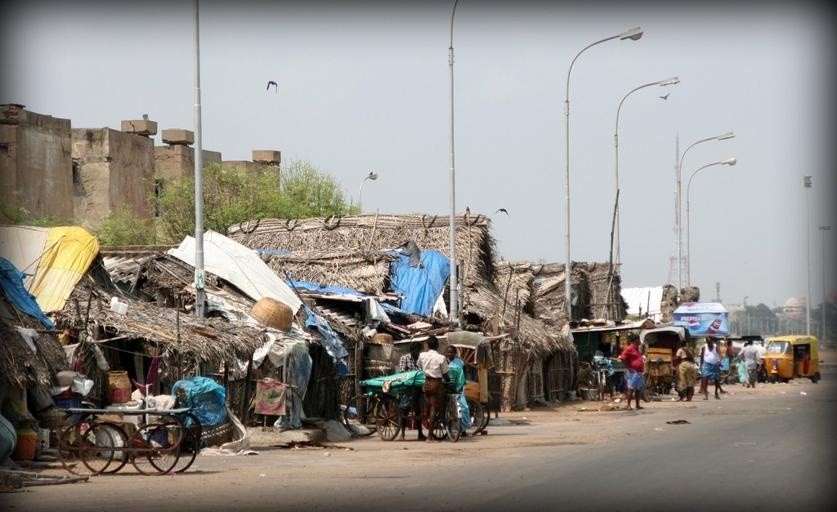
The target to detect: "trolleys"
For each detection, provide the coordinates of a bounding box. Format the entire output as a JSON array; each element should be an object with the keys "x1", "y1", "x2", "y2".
[{"x1": 54, "y1": 406, "x2": 198, "y2": 477}]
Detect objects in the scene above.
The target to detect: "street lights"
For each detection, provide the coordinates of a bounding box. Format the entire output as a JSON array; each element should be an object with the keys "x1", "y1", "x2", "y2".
[
  {"x1": 358, "y1": 170, "x2": 378, "y2": 213},
  {"x1": 563, "y1": 24, "x2": 646, "y2": 321},
  {"x1": 612, "y1": 75, "x2": 681, "y2": 274},
  {"x1": 802, "y1": 173, "x2": 812, "y2": 336},
  {"x1": 819, "y1": 224, "x2": 833, "y2": 349},
  {"x1": 677, "y1": 131, "x2": 738, "y2": 296}
]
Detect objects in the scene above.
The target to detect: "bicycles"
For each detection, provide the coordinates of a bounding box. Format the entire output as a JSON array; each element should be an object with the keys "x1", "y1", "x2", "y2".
[
  {"x1": 344, "y1": 378, "x2": 491, "y2": 442},
  {"x1": 589, "y1": 360, "x2": 607, "y2": 401},
  {"x1": 639, "y1": 358, "x2": 665, "y2": 403}
]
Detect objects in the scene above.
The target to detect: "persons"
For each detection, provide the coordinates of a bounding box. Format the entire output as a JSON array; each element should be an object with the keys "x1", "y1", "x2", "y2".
[
  {"x1": 399, "y1": 342, "x2": 426, "y2": 441},
  {"x1": 414, "y1": 336, "x2": 449, "y2": 443},
  {"x1": 445, "y1": 345, "x2": 470, "y2": 438},
  {"x1": 620, "y1": 333, "x2": 645, "y2": 409},
  {"x1": 674, "y1": 341, "x2": 697, "y2": 400},
  {"x1": 696, "y1": 336, "x2": 767, "y2": 401}
]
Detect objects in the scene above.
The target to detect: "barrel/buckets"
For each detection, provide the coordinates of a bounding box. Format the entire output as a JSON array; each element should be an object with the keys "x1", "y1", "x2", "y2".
[
  {"x1": 463, "y1": 383, "x2": 480, "y2": 399},
  {"x1": 105, "y1": 370, "x2": 133, "y2": 403}
]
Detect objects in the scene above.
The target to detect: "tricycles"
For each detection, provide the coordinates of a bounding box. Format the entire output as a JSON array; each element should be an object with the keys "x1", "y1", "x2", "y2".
[{"x1": 763, "y1": 333, "x2": 823, "y2": 383}]
